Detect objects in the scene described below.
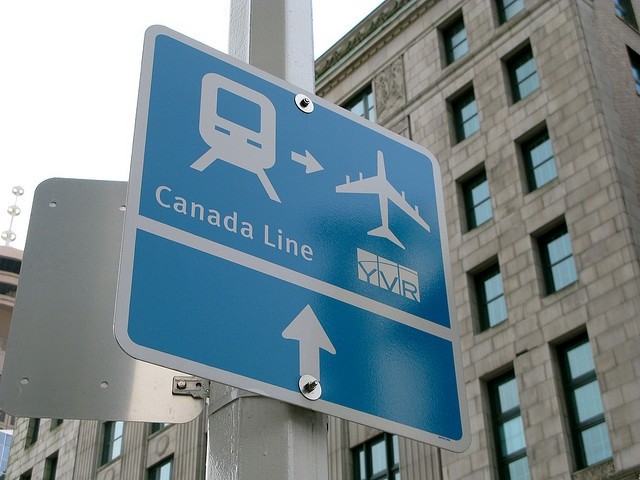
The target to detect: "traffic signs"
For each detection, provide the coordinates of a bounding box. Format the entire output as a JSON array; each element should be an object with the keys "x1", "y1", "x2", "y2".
[{"x1": 113, "y1": 25, "x2": 471, "y2": 452}]
